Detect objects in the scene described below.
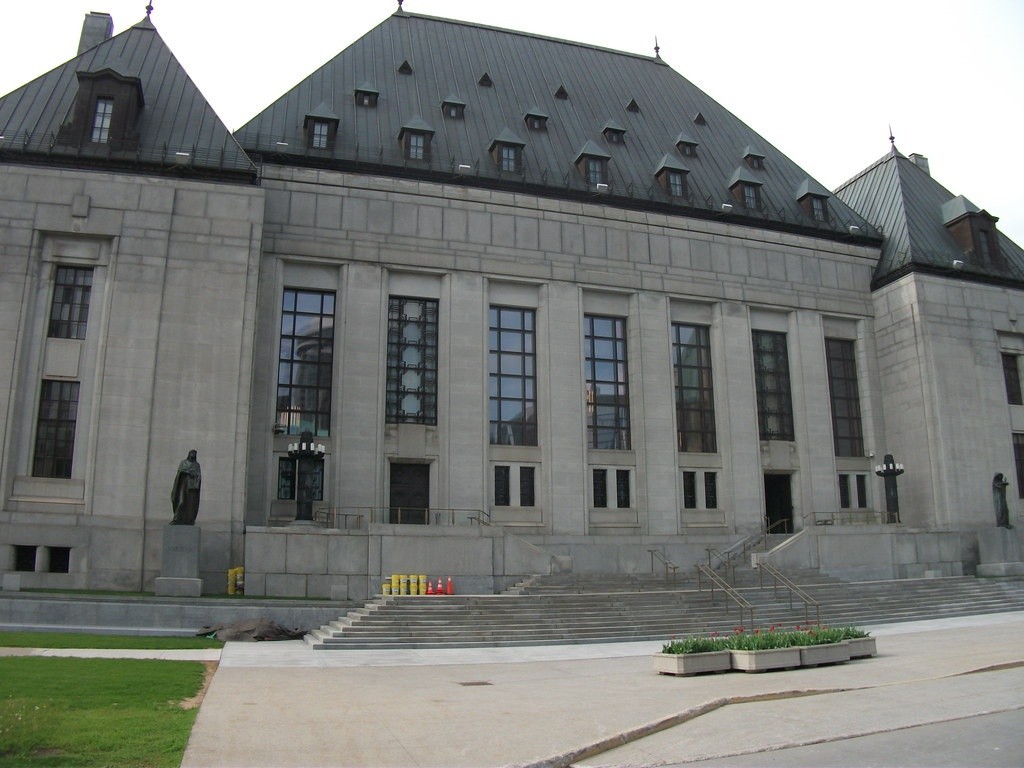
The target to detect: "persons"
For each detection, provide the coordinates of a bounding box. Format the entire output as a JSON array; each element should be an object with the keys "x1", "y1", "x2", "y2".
[
  {"x1": 172, "y1": 450, "x2": 201, "y2": 526},
  {"x1": 993, "y1": 473, "x2": 1016, "y2": 530}
]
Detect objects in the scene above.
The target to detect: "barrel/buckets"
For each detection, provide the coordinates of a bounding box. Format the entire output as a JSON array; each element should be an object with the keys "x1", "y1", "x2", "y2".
[
  {"x1": 419, "y1": 575, "x2": 427, "y2": 595},
  {"x1": 409, "y1": 574, "x2": 418, "y2": 595},
  {"x1": 392, "y1": 574, "x2": 399, "y2": 595},
  {"x1": 381, "y1": 583, "x2": 390, "y2": 595},
  {"x1": 400, "y1": 575, "x2": 408, "y2": 595},
  {"x1": 227, "y1": 566, "x2": 244, "y2": 594}
]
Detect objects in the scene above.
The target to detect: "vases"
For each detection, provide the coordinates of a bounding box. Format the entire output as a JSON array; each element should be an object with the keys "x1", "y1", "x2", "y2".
[
  {"x1": 799, "y1": 642, "x2": 850, "y2": 668},
  {"x1": 652, "y1": 650, "x2": 731, "y2": 676},
  {"x1": 842, "y1": 635, "x2": 877, "y2": 660},
  {"x1": 725, "y1": 646, "x2": 801, "y2": 673}
]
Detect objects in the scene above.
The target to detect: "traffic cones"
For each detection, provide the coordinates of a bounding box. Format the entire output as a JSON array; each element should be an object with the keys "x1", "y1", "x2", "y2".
[
  {"x1": 426, "y1": 577, "x2": 445, "y2": 595},
  {"x1": 445, "y1": 576, "x2": 455, "y2": 595}
]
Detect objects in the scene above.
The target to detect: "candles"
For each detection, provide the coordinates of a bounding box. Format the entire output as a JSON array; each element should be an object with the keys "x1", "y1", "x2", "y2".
[
  {"x1": 318, "y1": 444, "x2": 322, "y2": 451},
  {"x1": 896, "y1": 463, "x2": 899, "y2": 469},
  {"x1": 878, "y1": 465, "x2": 881, "y2": 471},
  {"x1": 293, "y1": 443, "x2": 299, "y2": 450},
  {"x1": 900, "y1": 464, "x2": 904, "y2": 469},
  {"x1": 302, "y1": 442, "x2": 306, "y2": 450},
  {"x1": 311, "y1": 443, "x2": 314, "y2": 450},
  {"x1": 321, "y1": 446, "x2": 325, "y2": 452},
  {"x1": 875, "y1": 466, "x2": 878, "y2": 472},
  {"x1": 889, "y1": 464, "x2": 893, "y2": 469},
  {"x1": 882, "y1": 464, "x2": 886, "y2": 470},
  {"x1": 288, "y1": 444, "x2": 293, "y2": 451}
]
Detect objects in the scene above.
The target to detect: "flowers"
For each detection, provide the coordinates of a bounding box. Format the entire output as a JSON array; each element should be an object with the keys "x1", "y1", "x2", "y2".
[
  {"x1": 787, "y1": 620, "x2": 845, "y2": 646},
  {"x1": 725, "y1": 624, "x2": 792, "y2": 651},
  {"x1": 662, "y1": 622, "x2": 725, "y2": 654},
  {"x1": 824, "y1": 615, "x2": 872, "y2": 639}
]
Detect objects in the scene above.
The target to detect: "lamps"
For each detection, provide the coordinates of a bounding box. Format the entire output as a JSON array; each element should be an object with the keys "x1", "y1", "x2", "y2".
[
  {"x1": 597, "y1": 184, "x2": 608, "y2": 192},
  {"x1": 173, "y1": 152, "x2": 192, "y2": 163},
  {"x1": 953, "y1": 259, "x2": 964, "y2": 269},
  {"x1": 850, "y1": 225, "x2": 859, "y2": 234},
  {"x1": 459, "y1": 164, "x2": 470, "y2": 173},
  {"x1": 276, "y1": 141, "x2": 288, "y2": 153},
  {"x1": 866, "y1": 451, "x2": 875, "y2": 460},
  {"x1": 721, "y1": 204, "x2": 733, "y2": 213}
]
